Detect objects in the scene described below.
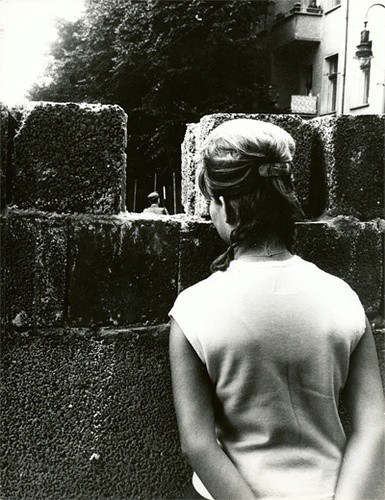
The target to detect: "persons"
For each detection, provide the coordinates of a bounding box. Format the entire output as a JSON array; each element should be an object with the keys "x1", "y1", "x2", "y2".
[
  {"x1": 143, "y1": 191, "x2": 168, "y2": 215},
  {"x1": 168, "y1": 118, "x2": 385, "y2": 500}
]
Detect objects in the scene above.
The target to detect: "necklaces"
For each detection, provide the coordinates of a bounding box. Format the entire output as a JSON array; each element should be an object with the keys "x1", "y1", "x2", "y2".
[{"x1": 235, "y1": 247, "x2": 289, "y2": 259}]
